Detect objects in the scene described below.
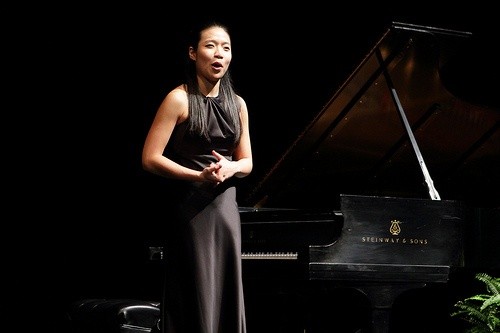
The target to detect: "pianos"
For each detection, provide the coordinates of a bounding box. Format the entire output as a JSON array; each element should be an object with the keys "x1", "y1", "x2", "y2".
[{"x1": 171, "y1": 20, "x2": 496, "y2": 316}]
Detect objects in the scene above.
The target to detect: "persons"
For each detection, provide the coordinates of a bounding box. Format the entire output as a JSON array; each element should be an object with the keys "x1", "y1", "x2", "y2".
[{"x1": 142, "y1": 21, "x2": 253, "y2": 333}]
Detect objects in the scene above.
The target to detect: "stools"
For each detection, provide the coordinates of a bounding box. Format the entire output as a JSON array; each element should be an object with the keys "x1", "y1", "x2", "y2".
[{"x1": 69, "y1": 298, "x2": 161, "y2": 333}]
